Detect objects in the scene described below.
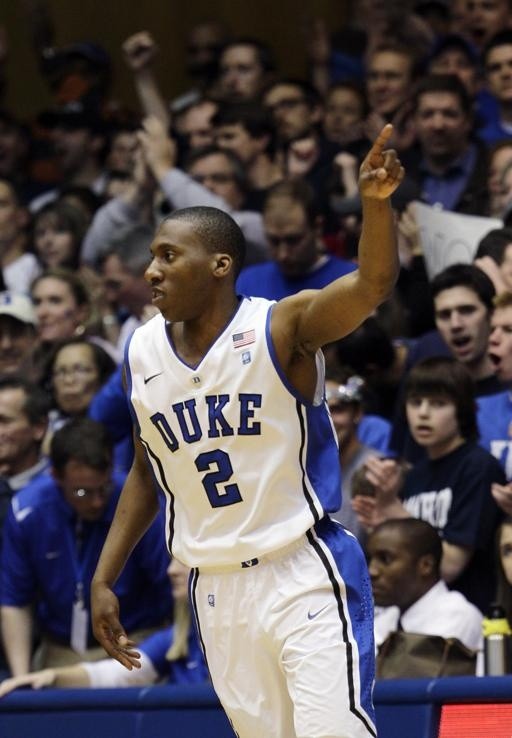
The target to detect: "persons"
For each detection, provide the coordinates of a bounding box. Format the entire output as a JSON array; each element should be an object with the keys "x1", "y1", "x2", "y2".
[
  {"x1": 1, "y1": 0, "x2": 512, "y2": 697},
  {"x1": 90, "y1": 119, "x2": 405, "y2": 738}
]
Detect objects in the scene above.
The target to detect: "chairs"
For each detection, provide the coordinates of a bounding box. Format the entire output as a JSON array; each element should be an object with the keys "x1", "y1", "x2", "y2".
[{"x1": 429, "y1": 699, "x2": 512, "y2": 738}]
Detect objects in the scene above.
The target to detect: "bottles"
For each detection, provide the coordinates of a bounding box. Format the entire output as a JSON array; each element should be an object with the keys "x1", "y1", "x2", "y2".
[{"x1": 483, "y1": 602, "x2": 508, "y2": 676}]
